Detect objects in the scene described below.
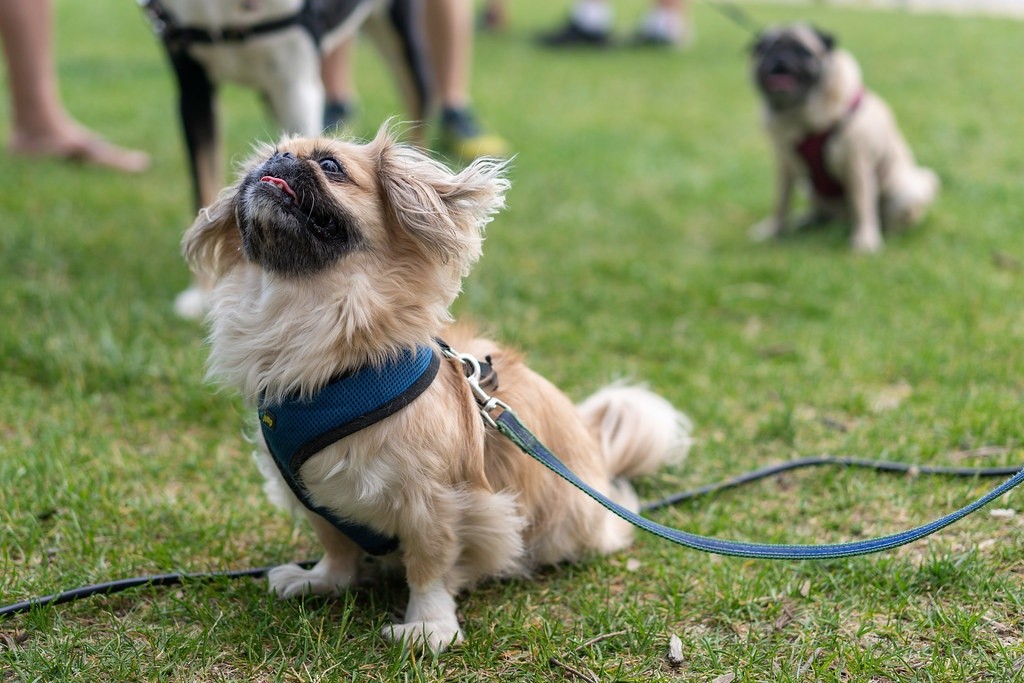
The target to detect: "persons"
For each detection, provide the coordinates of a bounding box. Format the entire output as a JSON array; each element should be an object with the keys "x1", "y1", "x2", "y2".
[
  {"x1": 323, "y1": 0, "x2": 508, "y2": 162},
  {"x1": 540, "y1": 0, "x2": 686, "y2": 49},
  {"x1": 0, "y1": 0, "x2": 148, "y2": 174}
]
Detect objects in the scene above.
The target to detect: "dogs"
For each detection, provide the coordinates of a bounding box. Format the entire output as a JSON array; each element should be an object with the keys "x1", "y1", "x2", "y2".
[
  {"x1": 179, "y1": 113, "x2": 693, "y2": 654},
  {"x1": 138, "y1": 1, "x2": 437, "y2": 320},
  {"x1": 744, "y1": 22, "x2": 940, "y2": 256}
]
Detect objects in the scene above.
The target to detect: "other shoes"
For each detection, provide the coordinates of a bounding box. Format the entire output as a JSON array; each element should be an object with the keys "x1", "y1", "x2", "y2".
[
  {"x1": 635, "y1": 24, "x2": 677, "y2": 42},
  {"x1": 533, "y1": 14, "x2": 609, "y2": 42}
]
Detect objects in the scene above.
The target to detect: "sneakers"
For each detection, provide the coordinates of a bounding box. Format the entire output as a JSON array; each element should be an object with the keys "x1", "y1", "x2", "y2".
[
  {"x1": 325, "y1": 97, "x2": 362, "y2": 133},
  {"x1": 443, "y1": 106, "x2": 511, "y2": 159}
]
